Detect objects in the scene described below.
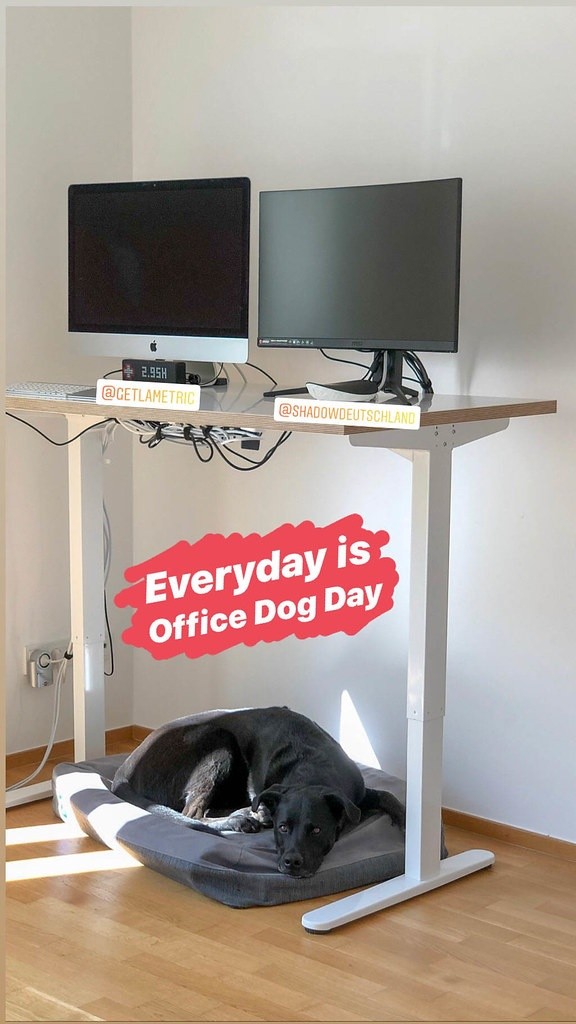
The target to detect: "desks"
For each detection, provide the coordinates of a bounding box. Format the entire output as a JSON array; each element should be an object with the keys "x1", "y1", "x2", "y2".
[{"x1": 7, "y1": 380, "x2": 557, "y2": 935}]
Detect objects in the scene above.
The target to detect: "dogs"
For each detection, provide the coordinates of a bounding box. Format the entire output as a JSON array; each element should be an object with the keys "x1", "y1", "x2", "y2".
[{"x1": 109, "y1": 704, "x2": 406, "y2": 881}]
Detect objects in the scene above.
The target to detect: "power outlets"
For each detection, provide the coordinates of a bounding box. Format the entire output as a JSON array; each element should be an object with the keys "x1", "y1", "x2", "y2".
[{"x1": 24, "y1": 640, "x2": 77, "y2": 674}]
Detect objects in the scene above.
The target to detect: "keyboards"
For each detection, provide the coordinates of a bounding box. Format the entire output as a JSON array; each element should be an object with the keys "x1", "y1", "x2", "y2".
[{"x1": 6, "y1": 382, "x2": 101, "y2": 403}]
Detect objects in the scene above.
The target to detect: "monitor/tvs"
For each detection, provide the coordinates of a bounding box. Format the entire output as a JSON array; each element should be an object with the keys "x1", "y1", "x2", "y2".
[
  {"x1": 67, "y1": 177, "x2": 253, "y2": 386},
  {"x1": 259, "y1": 177, "x2": 462, "y2": 405}
]
